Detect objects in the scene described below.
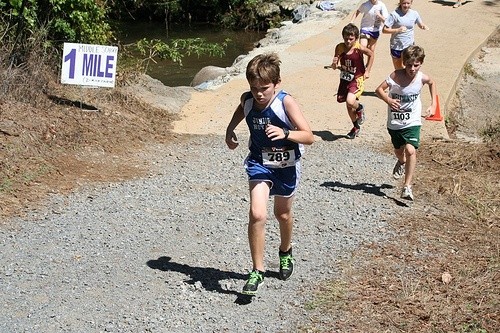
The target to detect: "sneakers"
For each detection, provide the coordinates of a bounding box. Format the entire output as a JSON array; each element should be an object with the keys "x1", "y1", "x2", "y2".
[
  {"x1": 358, "y1": 103, "x2": 366, "y2": 125},
  {"x1": 242, "y1": 269, "x2": 264, "y2": 296},
  {"x1": 346, "y1": 126, "x2": 360, "y2": 139},
  {"x1": 400, "y1": 184, "x2": 413, "y2": 201},
  {"x1": 278, "y1": 246, "x2": 293, "y2": 281},
  {"x1": 392, "y1": 160, "x2": 406, "y2": 180}
]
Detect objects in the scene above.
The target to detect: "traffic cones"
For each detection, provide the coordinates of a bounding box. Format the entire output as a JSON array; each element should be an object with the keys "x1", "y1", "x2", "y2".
[{"x1": 425, "y1": 95, "x2": 443, "y2": 121}]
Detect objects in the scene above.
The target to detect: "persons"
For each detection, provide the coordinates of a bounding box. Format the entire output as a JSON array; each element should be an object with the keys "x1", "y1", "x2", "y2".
[
  {"x1": 225, "y1": 53, "x2": 315, "y2": 294},
  {"x1": 376, "y1": 45, "x2": 438, "y2": 201},
  {"x1": 382, "y1": 0, "x2": 429, "y2": 71},
  {"x1": 349, "y1": 0, "x2": 389, "y2": 70},
  {"x1": 331, "y1": 22, "x2": 374, "y2": 139}
]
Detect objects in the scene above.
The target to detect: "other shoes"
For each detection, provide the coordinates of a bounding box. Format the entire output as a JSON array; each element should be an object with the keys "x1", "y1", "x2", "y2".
[{"x1": 452, "y1": 2, "x2": 462, "y2": 8}]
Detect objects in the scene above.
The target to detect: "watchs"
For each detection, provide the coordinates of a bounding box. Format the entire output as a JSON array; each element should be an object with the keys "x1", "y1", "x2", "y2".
[{"x1": 283, "y1": 126, "x2": 289, "y2": 138}]
